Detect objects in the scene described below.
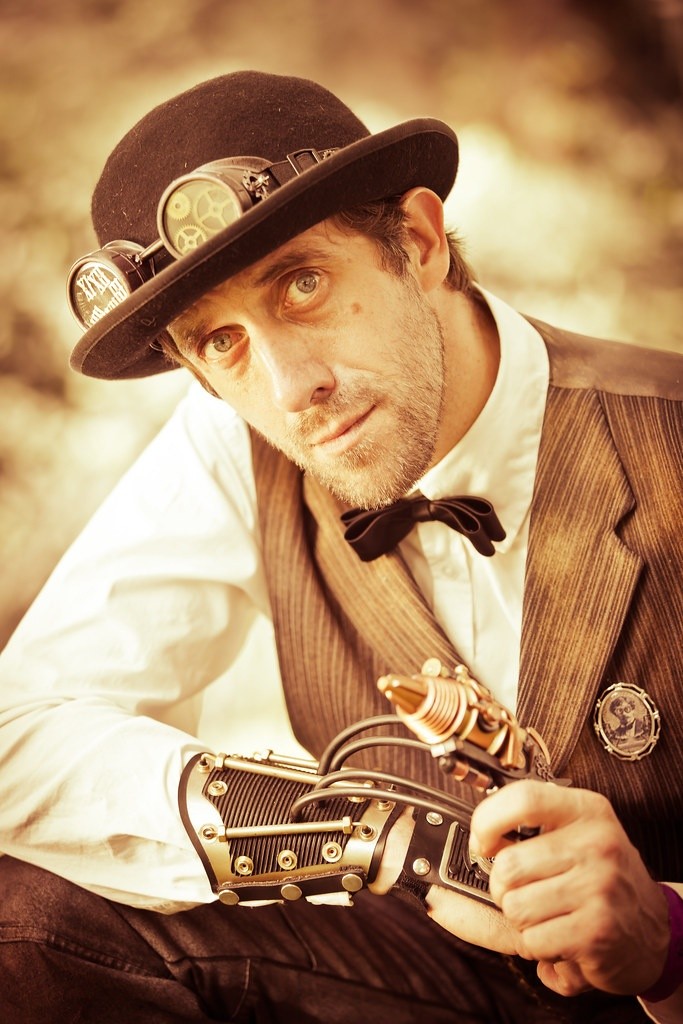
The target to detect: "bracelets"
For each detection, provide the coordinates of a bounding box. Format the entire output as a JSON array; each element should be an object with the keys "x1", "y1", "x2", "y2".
[{"x1": 638, "y1": 883, "x2": 683, "y2": 1003}]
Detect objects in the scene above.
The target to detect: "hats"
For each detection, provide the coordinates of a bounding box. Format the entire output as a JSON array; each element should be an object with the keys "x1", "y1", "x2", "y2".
[{"x1": 71, "y1": 70, "x2": 457, "y2": 378}]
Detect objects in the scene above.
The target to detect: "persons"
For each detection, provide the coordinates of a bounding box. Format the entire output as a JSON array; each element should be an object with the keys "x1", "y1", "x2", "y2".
[{"x1": 0, "y1": 69, "x2": 683, "y2": 1023}]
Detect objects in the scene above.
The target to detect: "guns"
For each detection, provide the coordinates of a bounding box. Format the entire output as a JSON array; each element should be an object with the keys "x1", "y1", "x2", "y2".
[{"x1": 372, "y1": 657, "x2": 553, "y2": 842}]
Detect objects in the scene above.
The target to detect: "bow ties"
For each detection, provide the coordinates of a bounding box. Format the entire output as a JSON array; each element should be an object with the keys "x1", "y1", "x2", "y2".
[{"x1": 341, "y1": 494, "x2": 505, "y2": 562}]
